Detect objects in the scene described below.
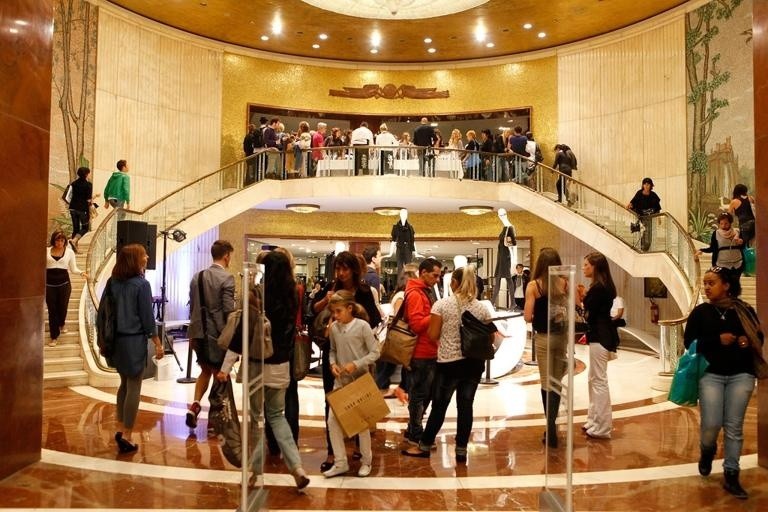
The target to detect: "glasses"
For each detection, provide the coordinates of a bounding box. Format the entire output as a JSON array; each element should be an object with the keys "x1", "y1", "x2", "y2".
[{"x1": 703, "y1": 266, "x2": 723, "y2": 274}]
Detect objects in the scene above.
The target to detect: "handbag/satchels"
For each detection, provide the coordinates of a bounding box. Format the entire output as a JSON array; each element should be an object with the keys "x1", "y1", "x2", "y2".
[
  {"x1": 458, "y1": 308, "x2": 497, "y2": 365},
  {"x1": 247, "y1": 283, "x2": 275, "y2": 362},
  {"x1": 743, "y1": 241, "x2": 757, "y2": 276},
  {"x1": 202, "y1": 331, "x2": 240, "y2": 368},
  {"x1": 291, "y1": 322, "x2": 311, "y2": 383},
  {"x1": 323, "y1": 369, "x2": 390, "y2": 440},
  {"x1": 311, "y1": 282, "x2": 336, "y2": 348},
  {"x1": 378, "y1": 314, "x2": 418, "y2": 369},
  {"x1": 667, "y1": 338, "x2": 709, "y2": 407},
  {"x1": 573, "y1": 357, "x2": 586, "y2": 376},
  {"x1": 98, "y1": 276, "x2": 118, "y2": 358}
]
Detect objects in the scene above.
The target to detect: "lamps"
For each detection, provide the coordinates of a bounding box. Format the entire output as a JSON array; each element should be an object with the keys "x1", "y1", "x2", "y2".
[{"x1": 159, "y1": 229, "x2": 187, "y2": 371}]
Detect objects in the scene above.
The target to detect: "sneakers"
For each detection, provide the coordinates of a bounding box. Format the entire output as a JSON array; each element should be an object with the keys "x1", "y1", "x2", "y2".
[
  {"x1": 452, "y1": 446, "x2": 468, "y2": 461},
  {"x1": 68, "y1": 239, "x2": 84, "y2": 254},
  {"x1": 58, "y1": 326, "x2": 67, "y2": 333},
  {"x1": 580, "y1": 422, "x2": 612, "y2": 442},
  {"x1": 403, "y1": 430, "x2": 437, "y2": 452},
  {"x1": 353, "y1": 450, "x2": 373, "y2": 477},
  {"x1": 48, "y1": 339, "x2": 57, "y2": 348},
  {"x1": 316, "y1": 456, "x2": 350, "y2": 479},
  {"x1": 239, "y1": 473, "x2": 263, "y2": 488},
  {"x1": 291, "y1": 467, "x2": 310, "y2": 491}
]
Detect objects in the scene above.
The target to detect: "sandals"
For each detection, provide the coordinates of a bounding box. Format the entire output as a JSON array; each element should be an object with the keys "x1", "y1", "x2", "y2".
[{"x1": 400, "y1": 444, "x2": 431, "y2": 459}]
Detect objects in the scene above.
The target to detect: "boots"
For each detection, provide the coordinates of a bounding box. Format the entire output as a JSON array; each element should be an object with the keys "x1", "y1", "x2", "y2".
[
  {"x1": 183, "y1": 400, "x2": 201, "y2": 430},
  {"x1": 696, "y1": 446, "x2": 716, "y2": 478},
  {"x1": 539, "y1": 386, "x2": 562, "y2": 450},
  {"x1": 720, "y1": 466, "x2": 750, "y2": 501}
]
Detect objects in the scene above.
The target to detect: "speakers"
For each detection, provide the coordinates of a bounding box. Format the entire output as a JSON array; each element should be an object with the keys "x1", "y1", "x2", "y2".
[{"x1": 117, "y1": 219, "x2": 147, "y2": 268}]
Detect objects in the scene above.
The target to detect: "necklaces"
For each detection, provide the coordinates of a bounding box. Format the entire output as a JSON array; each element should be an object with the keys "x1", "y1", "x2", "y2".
[{"x1": 712, "y1": 304, "x2": 732, "y2": 321}]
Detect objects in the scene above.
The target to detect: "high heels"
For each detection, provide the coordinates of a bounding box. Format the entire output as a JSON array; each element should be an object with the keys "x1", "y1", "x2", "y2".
[{"x1": 111, "y1": 430, "x2": 139, "y2": 454}]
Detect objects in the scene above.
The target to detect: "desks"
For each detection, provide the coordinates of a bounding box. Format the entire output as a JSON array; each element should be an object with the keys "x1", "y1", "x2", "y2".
[
  {"x1": 393, "y1": 159, "x2": 465, "y2": 181},
  {"x1": 316, "y1": 159, "x2": 378, "y2": 176}
]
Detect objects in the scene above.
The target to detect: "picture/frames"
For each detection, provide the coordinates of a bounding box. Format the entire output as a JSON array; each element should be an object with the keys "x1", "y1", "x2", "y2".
[{"x1": 644, "y1": 277, "x2": 668, "y2": 298}]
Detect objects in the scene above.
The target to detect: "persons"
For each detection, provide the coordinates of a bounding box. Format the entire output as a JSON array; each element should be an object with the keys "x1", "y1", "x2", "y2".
[
  {"x1": 682, "y1": 266, "x2": 764, "y2": 499},
  {"x1": 625, "y1": 178, "x2": 662, "y2": 252},
  {"x1": 611, "y1": 295, "x2": 627, "y2": 346},
  {"x1": 324, "y1": 122, "x2": 418, "y2": 176},
  {"x1": 581, "y1": 252, "x2": 617, "y2": 438},
  {"x1": 725, "y1": 185, "x2": 756, "y2": 276},
  {"x1": 187, "y1": 239, "x2": 309, "y2": 488},
  {"x1": 511, "y1": 265, "x2": 531, "y2": 311},
  {"x1": 307, "y1": 247, "x2": 510, "y2": 476},
  {"x1": 243, "y1": 118, "x2": 328, "y2": 184},
  {"x1": 552, "y1": 144, "x2": 578, "y2": 202},
  {"x1": 493, "y1": 209, "x2": 517, "y2": 311},
  {"x1": 694, "y1": 211, "x2": 745, "y2": 299},
  {"x1": 449, "y1": 126, "x2": 537, "y2": 190},
  {"x1": 61, "y1": 166, "x2": 101, "y2": 255},
  {"x1": 391, "y1": 209, "x2": 416, "y2": 272},
  {"x1": 96, "y1": 243, "x2": 166, "y2": 454},
  {"x1": 47, "y1": 230, "x2": 90, "y2": 347},
  {"x1": 103, "y1": 159, "x2": 130, "y2": 244},
  {"x1": 413, "y1": 118, "x2": 443, "y2": 177},
  {"x1": 525, "y1": 247, "x2": 580, "y2": 447}
]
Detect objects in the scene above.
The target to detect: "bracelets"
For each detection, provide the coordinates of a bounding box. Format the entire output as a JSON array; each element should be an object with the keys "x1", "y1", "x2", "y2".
[{"x1": 152, "y1": 343, "x2": 165, "y2": 352}]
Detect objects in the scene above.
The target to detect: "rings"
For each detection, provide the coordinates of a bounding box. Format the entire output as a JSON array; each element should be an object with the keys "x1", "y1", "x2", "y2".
[{"x1": 740, "y1": 342, "x2": 746, "y2": 345}]
